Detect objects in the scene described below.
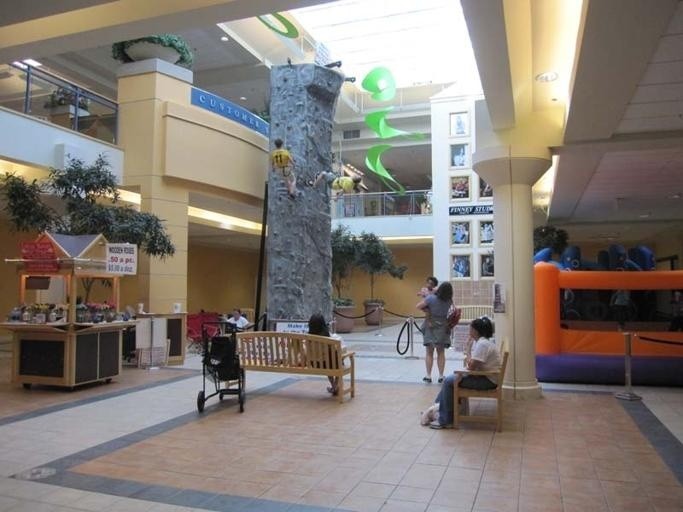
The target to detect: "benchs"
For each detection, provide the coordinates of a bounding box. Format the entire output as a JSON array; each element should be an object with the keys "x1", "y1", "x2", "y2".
[
  {"x1": 452, "y1": 336, "x2": 510, "y2": 432},
  {"x1": 218, "y1": 331, "x2": 355, "y2": 405},
  {"x1": 452, "y1": 305, "x2": 495, "y2": 325}
]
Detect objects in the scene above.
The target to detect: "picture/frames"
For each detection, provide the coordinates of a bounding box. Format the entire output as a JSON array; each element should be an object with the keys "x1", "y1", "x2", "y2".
[{"x1": 446, "y1": 111, "x2": 494, "y2": 280}]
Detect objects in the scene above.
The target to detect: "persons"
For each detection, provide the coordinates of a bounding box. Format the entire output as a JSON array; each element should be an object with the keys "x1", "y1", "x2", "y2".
[
  {"x1": 269, "y1": 138, "x2": 297, "y2": 199},
  {"x1": 76, "y1": 294, "x2": 88, "y2": 319},
  {"x1": 416, "y1": 276, "x2": 438, "y2": 328},
  {"x1": 416, "y1": 282, "x2": 453, "y2": 383},
  {"x1": 226, "y1": 307, "x2": 250, "y2": 329},
  {"x1": 303, "y1": 313, "x2": 344, "y2": 396},
  {"x1": 307, "y1": 171, "x2": 361, "y2": 202},
  {"x1": 608, "y1": 290, "x2": 631, "y2": 331},
  {"x1": 427, "y1": 316, "x2": 503, "y2": 430}
]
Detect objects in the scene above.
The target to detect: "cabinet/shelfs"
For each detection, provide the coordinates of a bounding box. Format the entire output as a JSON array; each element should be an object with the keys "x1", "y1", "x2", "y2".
[{"x1": 0, "y1": 230, "x2": 140, "y2": 392}]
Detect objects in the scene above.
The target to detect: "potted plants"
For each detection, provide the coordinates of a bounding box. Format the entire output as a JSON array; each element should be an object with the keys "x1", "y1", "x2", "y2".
[
  {"x1": 43, "y1": 85, "x2": 91, "y2": 130},
  {"x1": 111, "y1": 33, "x2": 194, "y2": 69},
  {"x1": 353, "y1": 230, "x2": 395, "y2": 325},
  {"x1": 330, "y1": 223, "x2": 360, "y2": 333}
]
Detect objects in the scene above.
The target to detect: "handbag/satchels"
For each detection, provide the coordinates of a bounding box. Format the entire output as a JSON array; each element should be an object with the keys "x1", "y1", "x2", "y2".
[{"x1": 448, "y1": 309, "x2": 461, "y2": 328}]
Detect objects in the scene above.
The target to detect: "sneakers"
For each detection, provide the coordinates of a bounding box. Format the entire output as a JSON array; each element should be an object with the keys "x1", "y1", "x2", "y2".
[
  {"x1": 423, "y1": 377, "x2": 443, "y2": 383},
  {"x1": 327, "y1": 386, "x2": 338, "y2": 395},
  {"x1": 429, "y1": 421, "x2": 453, "y2": 429}
]
